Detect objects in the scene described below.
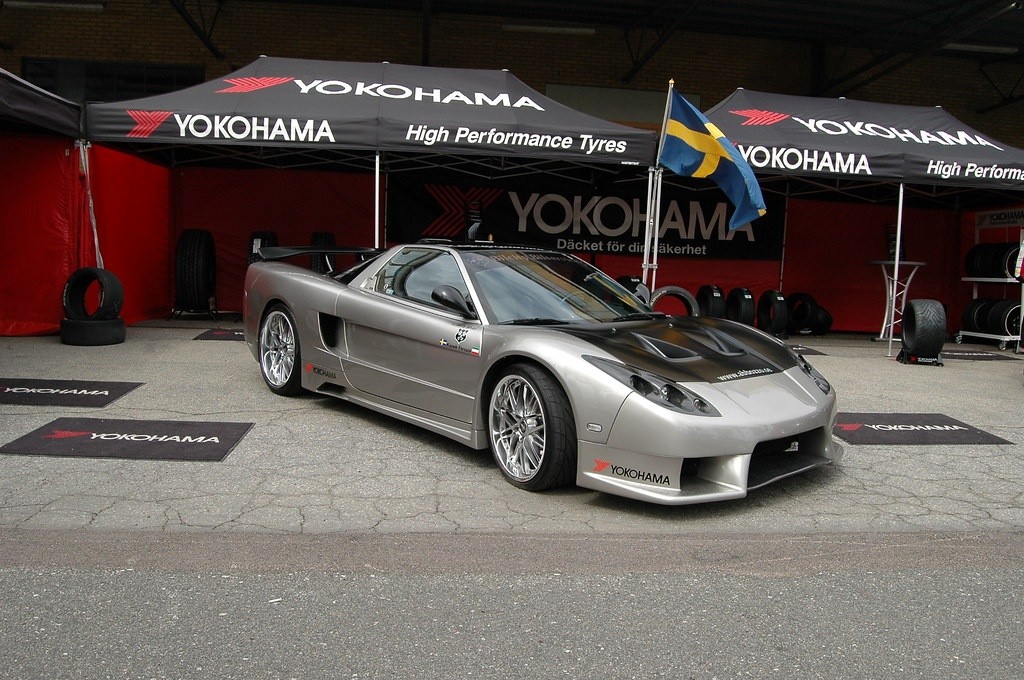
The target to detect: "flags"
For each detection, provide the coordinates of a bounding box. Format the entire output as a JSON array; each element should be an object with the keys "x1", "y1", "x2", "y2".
[{"x1": 661, "y1": 90, "x2": 766, "y2": 228}]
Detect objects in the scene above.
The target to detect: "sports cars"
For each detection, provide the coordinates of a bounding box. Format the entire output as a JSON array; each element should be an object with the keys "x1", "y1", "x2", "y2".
[{"x1": 241, "y1": 222, "x2": 844, "y2": 508}]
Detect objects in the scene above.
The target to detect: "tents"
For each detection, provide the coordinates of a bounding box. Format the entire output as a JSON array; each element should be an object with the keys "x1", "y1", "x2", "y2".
[
  {"x1": 651, "y1": 88, "x2": 1024, "y2": 357},
  {"x1": 0, "y1": 68, "x2": 99, "y2": 269},
  {"x1": 81, "y1": 55, "x2": 656, "y2": 284}
]
